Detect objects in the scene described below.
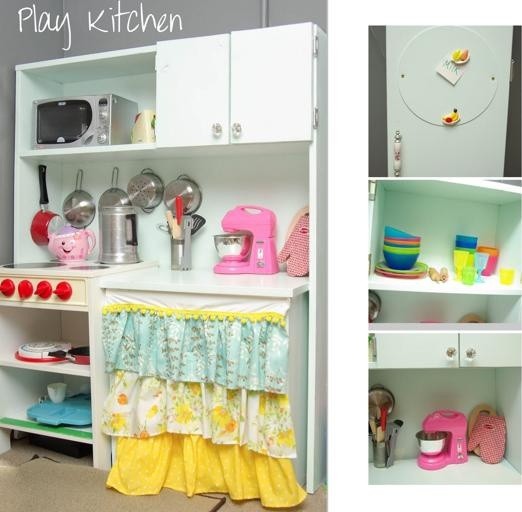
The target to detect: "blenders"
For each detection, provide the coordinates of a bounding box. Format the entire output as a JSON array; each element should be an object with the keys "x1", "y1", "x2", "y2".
[
  {"x1": 213, "y1": 206, "x2": 280, "y2": 275},
  {"x1": 416, "y1": 411, "x2": 469, "y2": 470}
]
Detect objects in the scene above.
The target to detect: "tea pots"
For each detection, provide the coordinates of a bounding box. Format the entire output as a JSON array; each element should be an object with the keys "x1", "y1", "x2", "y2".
[{"x1": 47, "y1": 224, "x2": 96, "y2": 262}]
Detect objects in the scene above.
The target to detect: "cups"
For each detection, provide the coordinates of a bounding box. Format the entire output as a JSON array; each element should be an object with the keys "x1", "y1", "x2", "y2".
[
  {"x1": 454, "y1": 234, "x2": 477, "y2": 285},
  {"x1": 373, "y1": 442, "x2": 388, "y2": 468},
  {"x1": 478, "y1": 247, "x2": 501, "y2": 276},
  {"x1": 47, "y1": 382, "x2": 67, "y2": 404},
  {"x1": 499, "y1": 269, "x2": 516, "y2": 286},
  {"x1": 170, "y1": 240, "x2": 194, "y2": 271}
]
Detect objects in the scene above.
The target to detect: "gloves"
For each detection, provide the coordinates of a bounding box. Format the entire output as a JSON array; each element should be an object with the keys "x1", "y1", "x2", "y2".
[
  {"x1": 467, "y1": 416, "x2": 506, "y2": 464},
  {"x1": 279, "y1": 214, "x2": 309, "y2": 279}
]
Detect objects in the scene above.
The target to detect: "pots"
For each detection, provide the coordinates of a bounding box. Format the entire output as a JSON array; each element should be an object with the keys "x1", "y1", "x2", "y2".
[
  {"x1": 48, "y1": 346, "x2": 89, "y2": 365},
  {"x1": 98, "y1": 167, "x2": 133, "y2": 207},
  {"x1": 62, "y1": 170, "x2": 96, "y2": 227},
  {"x1": 164, "y1": 173, "x2": 202, "y2": 217},
  {"x1": 369, "y1": 384, "x2": 394, "y2": 421},
  {"x1": 32, "y1": 164, "x2": 63, "y2": 246},
  {"x1": 369, "y1": 289, "x2": 381, "y2": 323}
]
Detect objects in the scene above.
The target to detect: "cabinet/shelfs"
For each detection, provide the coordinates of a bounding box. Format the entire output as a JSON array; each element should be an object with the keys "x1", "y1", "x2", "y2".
[
  {"x1": 369, "y1": 178, "x2": 520, "y2": 486},
  {"x1": 0, "y1": 302, "x2": 112, "y2": 469},
  {"x1": 11, "y1": 21, "x2": 327, "y2": 284}
]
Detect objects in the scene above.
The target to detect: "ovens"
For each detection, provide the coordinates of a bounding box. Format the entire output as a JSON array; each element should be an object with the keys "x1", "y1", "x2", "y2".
[{"x1": 30, "y1": 92, "x2": 139, "y2": 151}]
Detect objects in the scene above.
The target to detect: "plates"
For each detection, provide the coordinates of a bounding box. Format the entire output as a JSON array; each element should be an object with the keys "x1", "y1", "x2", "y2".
[{"x1": 374, "y1": 261, "x2": 427, "y2": 278}]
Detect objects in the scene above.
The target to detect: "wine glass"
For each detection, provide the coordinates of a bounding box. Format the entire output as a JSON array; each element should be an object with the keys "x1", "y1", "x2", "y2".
[{"x1": 474, "y1": 252, "x2": 489, "y2": 282}]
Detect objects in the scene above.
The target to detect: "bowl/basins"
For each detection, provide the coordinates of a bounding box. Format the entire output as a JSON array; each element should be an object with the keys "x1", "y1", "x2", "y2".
[{"x1": 381, "y1": 226, "x2": 421, "y2": 269}]
